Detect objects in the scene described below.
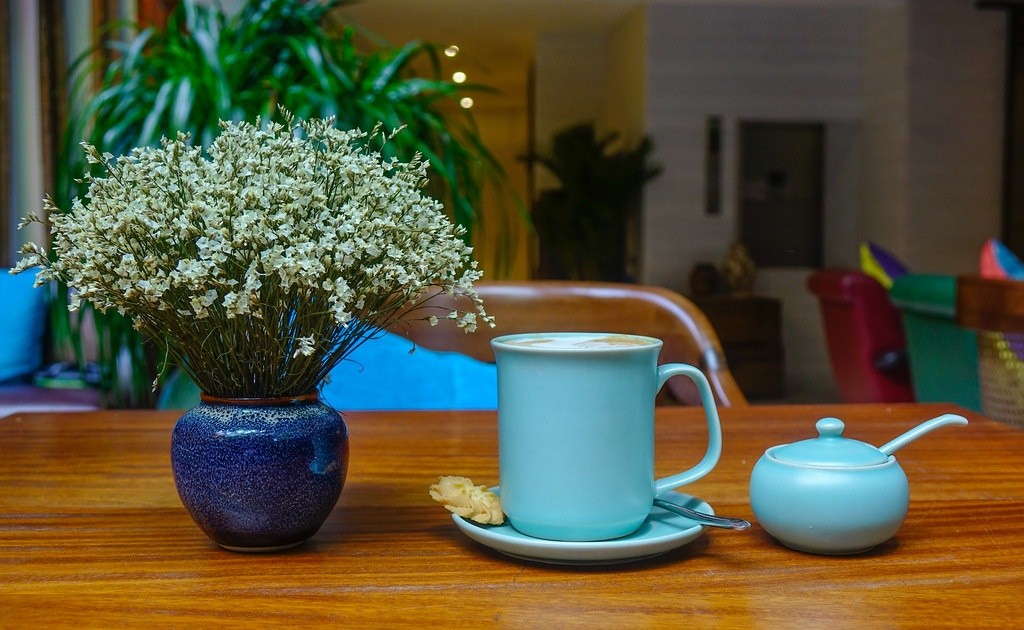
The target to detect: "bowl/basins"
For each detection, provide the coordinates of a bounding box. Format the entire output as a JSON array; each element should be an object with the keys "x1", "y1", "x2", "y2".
[{"x1": 749, "y1": 416, "x2": 910, "y2": 555}]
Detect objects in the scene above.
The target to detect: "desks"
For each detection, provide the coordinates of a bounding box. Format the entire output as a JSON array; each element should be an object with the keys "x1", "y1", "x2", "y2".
[{"x1": 0, "y1": 400, "x2": 1024, "y2": 629}]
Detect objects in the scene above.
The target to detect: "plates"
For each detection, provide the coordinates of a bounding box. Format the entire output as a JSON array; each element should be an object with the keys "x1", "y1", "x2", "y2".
[{"x1": 453, "y1": 486, "x2": 716, "y2": 564}]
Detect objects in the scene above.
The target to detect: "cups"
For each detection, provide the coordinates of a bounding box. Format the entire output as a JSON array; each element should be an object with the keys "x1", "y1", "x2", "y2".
[{"x1": 491, "y1": 330, "x2": 721, "y2": 541}]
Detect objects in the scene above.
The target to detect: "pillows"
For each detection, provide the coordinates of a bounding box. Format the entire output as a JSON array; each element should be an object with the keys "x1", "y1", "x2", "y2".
[
  {"x1": 290, "y1": 298, "x2": 497, "y2": 410},
  {"x1": 859, "y1": 237, "x2": 908, "y2": 287},
  {"x1": 979, "y1": 239, "x2": 1024, "y2": 279},
  {"x1": 0, "y1": 265, "x2": 50, "y2": 379}
]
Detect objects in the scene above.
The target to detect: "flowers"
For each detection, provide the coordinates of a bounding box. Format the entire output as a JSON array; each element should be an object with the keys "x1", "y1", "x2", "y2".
[{"x1": 6, "y1": 102, "x2": 495, "y2": 392}]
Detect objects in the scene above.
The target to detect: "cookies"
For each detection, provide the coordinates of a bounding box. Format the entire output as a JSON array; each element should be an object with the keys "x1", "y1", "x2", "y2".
[{"x1": 429, "y1": 474, "x2": 504, "y2": 525}]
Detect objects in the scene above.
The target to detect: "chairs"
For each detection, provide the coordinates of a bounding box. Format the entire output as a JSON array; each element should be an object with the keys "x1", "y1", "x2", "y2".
[
  {"x1": 807, "y1": 267, "x2": 1024, "y2": 431},
  {"x1": 346, "y1": 282, "x2": 785, "y2": 412}
]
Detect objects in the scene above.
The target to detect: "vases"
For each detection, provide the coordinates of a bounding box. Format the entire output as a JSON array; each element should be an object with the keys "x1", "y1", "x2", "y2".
[{"x1": 168, "y1": 392, "x2": 349, "y2": 553}]
